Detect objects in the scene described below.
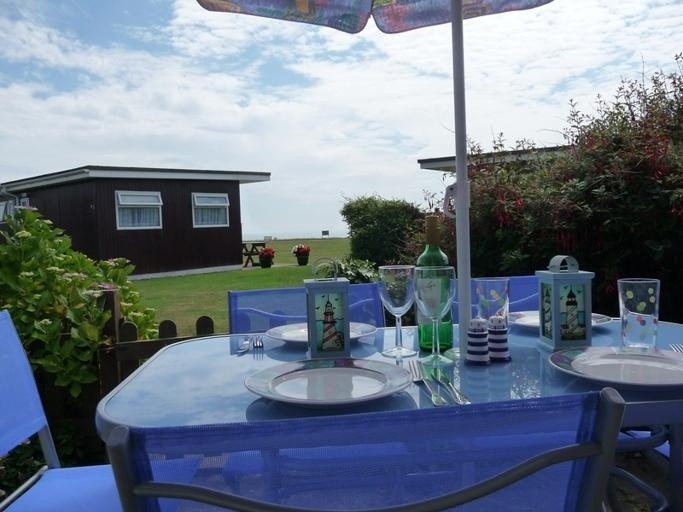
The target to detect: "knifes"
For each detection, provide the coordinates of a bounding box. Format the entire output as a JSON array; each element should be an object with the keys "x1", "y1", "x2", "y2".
[
  {"x1": 431, "y1": 368, "x2": 471, "y2": 406},
  {"x1": 236, "y1": 335, "x2": 252, "y2": 354}
]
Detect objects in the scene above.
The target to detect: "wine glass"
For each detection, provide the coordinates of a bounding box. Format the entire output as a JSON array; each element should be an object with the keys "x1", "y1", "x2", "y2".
[
  {"x1": 378, "y1": 263, "x2": 417, "y2": 359},
  {"x1": 413, "y1": 265, "x2": 457, "y2": 367}
]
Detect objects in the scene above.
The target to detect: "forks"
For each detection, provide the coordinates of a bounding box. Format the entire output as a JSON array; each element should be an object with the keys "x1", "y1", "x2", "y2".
[
  {"x1": 407, "y1": 360, "x2": 450, "y2": 406},
  {"x1": 250, "y1": 335, "x2": 265, "y2": 349}
]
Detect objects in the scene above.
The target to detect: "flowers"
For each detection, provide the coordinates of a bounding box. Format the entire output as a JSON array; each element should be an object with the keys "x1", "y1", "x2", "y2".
[
  {"x1": 259, "y1": 248, "x2": 274, "y2": 256},
  {"x1": 292, "y1": 244, "x2": 310, "y2": 255}
]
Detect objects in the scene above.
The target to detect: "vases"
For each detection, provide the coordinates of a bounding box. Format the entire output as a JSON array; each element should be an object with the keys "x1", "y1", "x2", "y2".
[
  {"x1": 296, "y1": 252, "x2": 309, "y2": 265},
  {"x1": 260, "y1": 255, "x2": 274, "y2": 268}
]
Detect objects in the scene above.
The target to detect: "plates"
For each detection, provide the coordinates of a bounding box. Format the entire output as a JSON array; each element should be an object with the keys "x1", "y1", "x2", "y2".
[
  {"x1": 265, "y1": 319, "x2": 377, "y2": 344},
  {"x1": 243, "y1": 356, "x2": 414, "y2": 409},
  {"x1": 549, "y1": 346, "x2": 683, "y2": 393}
]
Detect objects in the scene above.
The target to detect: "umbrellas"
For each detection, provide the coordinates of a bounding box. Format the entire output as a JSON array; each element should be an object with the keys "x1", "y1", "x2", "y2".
[{"x1": 196, "y1": 0, "x2": 552, "y2": 359}]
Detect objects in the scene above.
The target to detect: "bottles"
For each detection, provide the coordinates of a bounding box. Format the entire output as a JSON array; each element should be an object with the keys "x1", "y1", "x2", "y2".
[{"x1": 417, "y1": 215, "x2": 455, "y2": 351}]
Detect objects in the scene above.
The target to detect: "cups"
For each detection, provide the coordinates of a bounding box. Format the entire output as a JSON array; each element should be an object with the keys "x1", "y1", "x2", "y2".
[
  {"x1": 466, "y1": 278, "x2": 513, "y2": 367},
  {"x1": 618, "y1": 278, "x2": 660, "y2": 349}
]
